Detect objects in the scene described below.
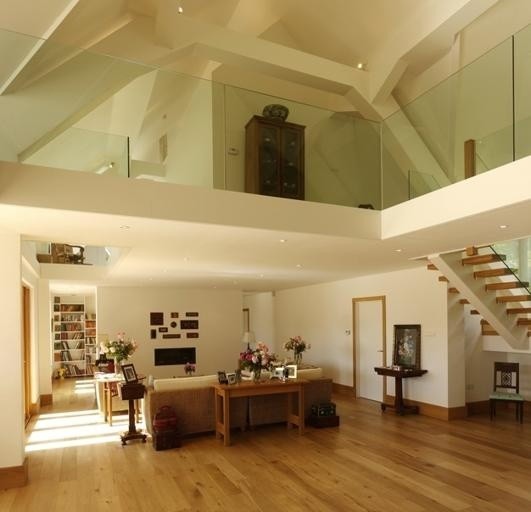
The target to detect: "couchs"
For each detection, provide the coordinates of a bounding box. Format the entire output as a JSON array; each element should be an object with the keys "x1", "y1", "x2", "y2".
[
  {"x1": 141, "y1": 366, "x2": 333, "y2": 436},
  {"x1": 92, "y1": 371, "x2": 141, "y2": 417}
]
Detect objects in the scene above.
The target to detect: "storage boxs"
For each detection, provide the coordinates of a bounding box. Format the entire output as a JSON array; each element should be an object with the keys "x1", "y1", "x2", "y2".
[
  {"x1": 310, "y1": 413, "x2": 340, "y2": 429},
  {"x1": 152, "y1": 429, "x2": 185, "y2": 450}
]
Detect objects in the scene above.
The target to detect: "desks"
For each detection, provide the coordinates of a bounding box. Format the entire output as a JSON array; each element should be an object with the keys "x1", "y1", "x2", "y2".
[
  {"x1": 375, "y1": 363, "x2": 431, "y2": 414},
  {"x1": 211, "y1": 378, "x2": 311, "y2": 446},
  {"x1": 95, "y1": 372, "x2": 149, "y2": 426}
]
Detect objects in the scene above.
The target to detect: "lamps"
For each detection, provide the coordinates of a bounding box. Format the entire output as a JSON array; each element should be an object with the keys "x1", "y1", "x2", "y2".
[{"x1": 242, "y1": 332, "x2": 257, "y2": 350}]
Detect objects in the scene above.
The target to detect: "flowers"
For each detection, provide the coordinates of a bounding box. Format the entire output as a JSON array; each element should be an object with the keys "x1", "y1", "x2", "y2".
[
  {"x1": 285, "y1": 334, "x2": 313, "y2": 353},
  {"x1": 184, "y1": 362, "x2": 198, "y2": 376},
  {"x1": 96, "y1": 331, "x2": 139, "y2": 361},
  {"x1": 239, "y1": 336, "x2": 277, "y2": 369}
]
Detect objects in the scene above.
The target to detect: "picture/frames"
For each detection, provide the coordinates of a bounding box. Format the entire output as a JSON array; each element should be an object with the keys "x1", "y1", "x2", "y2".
[
  {"x1": 284, "y1": 364, "x2": 297, "y2": 379},
  {"x1": 393, "y1": 323, "x2": 422, "y2": 370},
  {"x1": 217, "y1": 370, "x2": 227, "y2": 386},
  {"x1": 121, "y1": 362, "x2": 139, "y2": 384},
  {"x1": 226, "y1": 373, "x2": 236, "y2": 384},
  {"x1": 148, "y1": 310, "x2": 200, "y2": 340},
  {"x1": 269, "y1": 367, "x2": 283, "y2": 380}
]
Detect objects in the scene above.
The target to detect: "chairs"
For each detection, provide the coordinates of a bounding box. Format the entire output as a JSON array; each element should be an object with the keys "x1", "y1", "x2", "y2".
[{"x1": 489, "y1": 359, "x2": 527, "y2": 424}]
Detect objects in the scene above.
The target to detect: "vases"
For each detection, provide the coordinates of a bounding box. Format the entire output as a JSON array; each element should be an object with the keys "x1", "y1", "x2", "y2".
[
  {"x1": 251, "y1": 366, "x2": 265, "y2": 385},
  {"x1": 294, "y1": 350, "x2": 304, "y2": 366},
  {"x1": 114, "y1": 355, "x2": 126, "y2": 378}
]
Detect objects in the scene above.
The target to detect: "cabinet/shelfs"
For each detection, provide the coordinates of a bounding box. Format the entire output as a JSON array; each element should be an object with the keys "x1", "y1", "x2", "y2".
[
  {"x1": 54, "y1": 302, "x2": 86, "y2": 377},
  {"x1": 244, "y1": 111, "x2": 308, "y2": 198},
  {"x1": 85, "y1": 319, "x2": 98, "y2": 376}
]
